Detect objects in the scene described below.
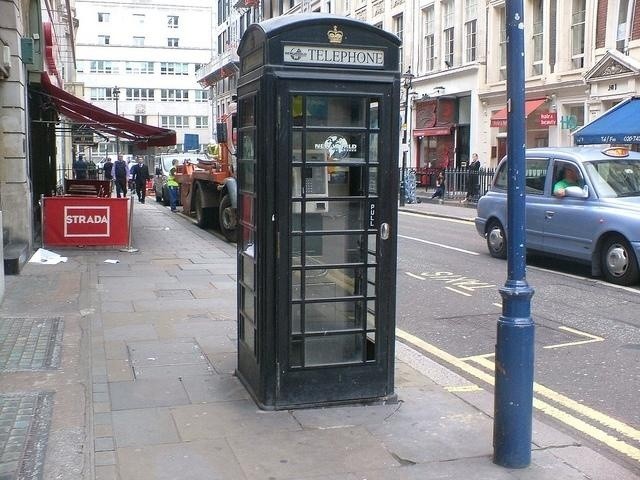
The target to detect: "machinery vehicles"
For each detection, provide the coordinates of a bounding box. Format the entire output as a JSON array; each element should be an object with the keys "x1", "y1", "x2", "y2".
[{"x1": 174, "y1": 101, "x2": 239, "y2": 247}]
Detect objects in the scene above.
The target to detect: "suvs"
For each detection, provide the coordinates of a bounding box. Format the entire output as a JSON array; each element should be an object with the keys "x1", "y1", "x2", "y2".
[
  {"x1": 154, "y1": 152, "x2": 213, "y2": 206},
  {"x1": 475, "y1": 144, "x2": 640, "y2": 284}
]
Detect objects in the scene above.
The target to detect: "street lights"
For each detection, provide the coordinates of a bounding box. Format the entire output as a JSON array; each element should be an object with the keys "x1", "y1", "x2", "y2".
[
  {"x1": 112, "y1": 86, "x2": 121, "y2": 162},
  {"x1": 400, "y1": 65, "x2": 413, "y2": 208}
]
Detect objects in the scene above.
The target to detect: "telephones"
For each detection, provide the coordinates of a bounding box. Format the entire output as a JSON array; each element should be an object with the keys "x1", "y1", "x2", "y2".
[{"x1": 292, "y1": 149, "x2": 328, "y2": 214}]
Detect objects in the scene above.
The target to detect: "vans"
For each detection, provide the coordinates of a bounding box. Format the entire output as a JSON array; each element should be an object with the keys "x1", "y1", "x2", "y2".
[{"x1": 96, "y1": 154, "x2": 133, "y2": 174}]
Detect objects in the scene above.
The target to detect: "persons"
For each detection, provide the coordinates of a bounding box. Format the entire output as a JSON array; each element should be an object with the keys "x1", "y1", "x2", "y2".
[
  {"x1": 103, "y1": 158, "x2": 115, "y2": 180},
  {"x1": 114, "y1": 153, "x2": 130, "y2": 198},
  {"x1": 74, "y1": 155, "x2": 89, "y2": 179},
  {"x1": 553, "y1": 162, "x2": 584, "y2": 197},
  {"x1": 430, "y1": 172, "x2": 445, "y2": 199},
  {"x1": 465, "y1": 153, "x2": 480, "y2": 200},
  {"x1": 131, "y1": 155, "x2": 150, "y2": 204},
  {"x1": 166, "y1": 159, "x2": 183, "y2": 212}
]
